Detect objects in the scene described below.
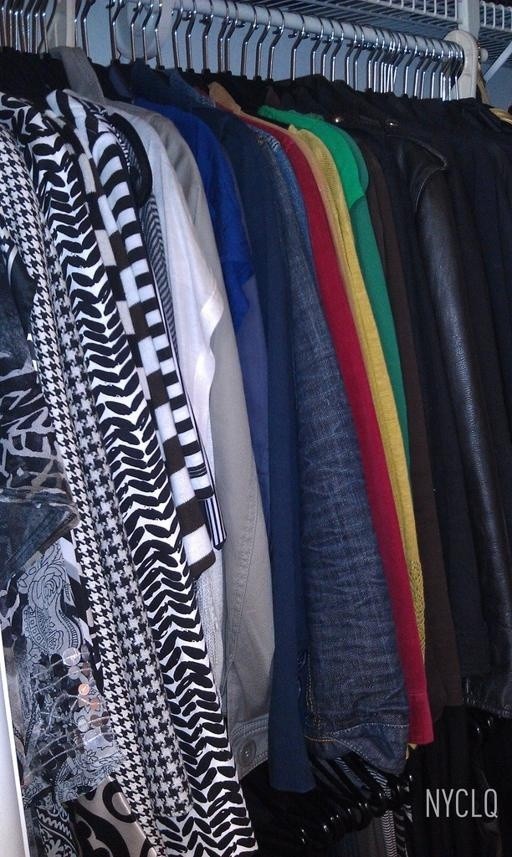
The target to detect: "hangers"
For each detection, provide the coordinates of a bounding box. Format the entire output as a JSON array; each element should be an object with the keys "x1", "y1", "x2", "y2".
[{"x1": 0, "y1": 0, "x2": 512, "y2": 132}]
